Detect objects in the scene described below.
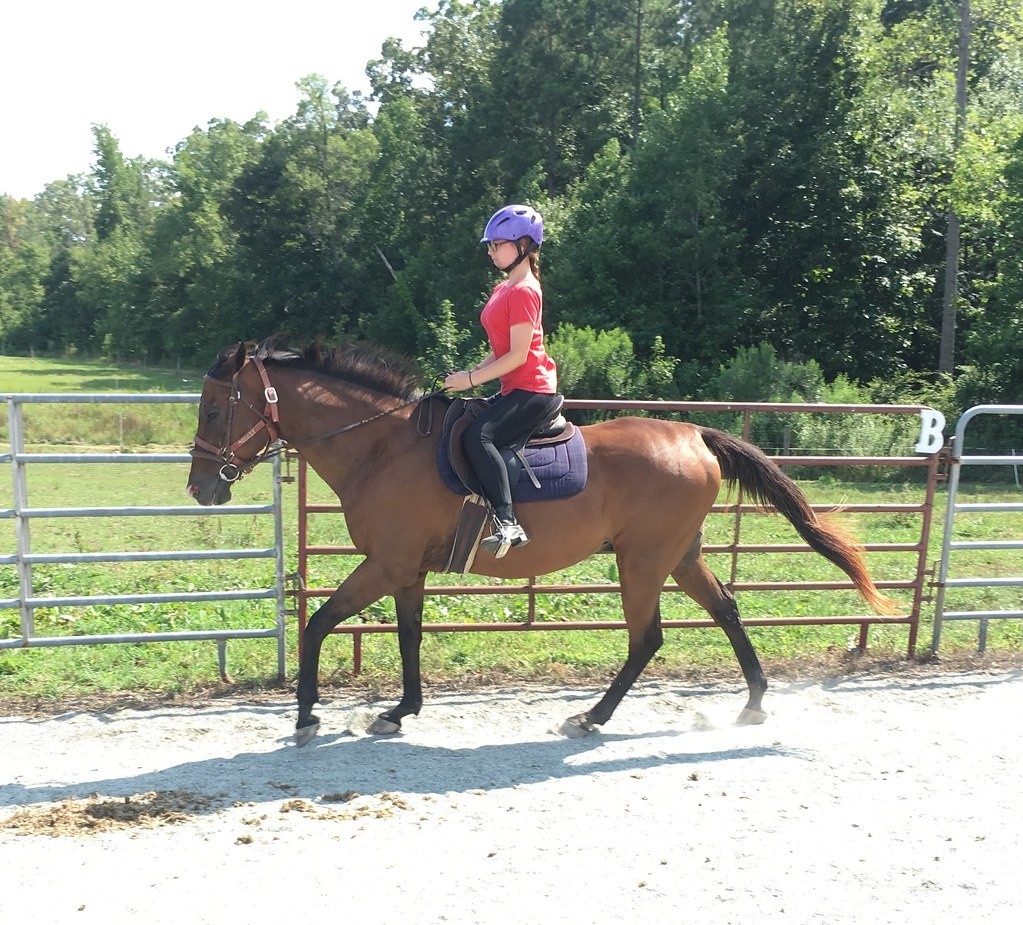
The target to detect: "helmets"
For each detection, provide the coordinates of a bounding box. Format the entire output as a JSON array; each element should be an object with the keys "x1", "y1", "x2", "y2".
[{"x1": 479, "y1": 205, "x2": 542, "y2": 246}]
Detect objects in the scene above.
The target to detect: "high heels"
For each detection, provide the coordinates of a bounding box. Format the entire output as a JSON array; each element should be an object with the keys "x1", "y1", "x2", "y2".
[{"x1": 481, "y1": 524, "x2": 529, "y2": 551}]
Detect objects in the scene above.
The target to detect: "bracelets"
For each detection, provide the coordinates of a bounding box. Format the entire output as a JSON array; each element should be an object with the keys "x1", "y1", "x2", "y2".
[
  {"x1": 468, "y1": 370, "x2": 470, "y2": 373},
  {"x1": 469, "y1": 372, "x2": 477, "y2": 387}
]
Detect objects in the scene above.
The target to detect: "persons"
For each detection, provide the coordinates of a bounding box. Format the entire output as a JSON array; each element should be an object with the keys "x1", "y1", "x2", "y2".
[{"x1": 443, "y1": 205, "x2": 557, "y2": 553}]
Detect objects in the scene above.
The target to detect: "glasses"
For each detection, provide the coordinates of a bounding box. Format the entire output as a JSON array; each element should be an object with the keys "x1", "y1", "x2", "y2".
[{"x1": 487, "y1": 241, "x2": 513, "y2": 252}]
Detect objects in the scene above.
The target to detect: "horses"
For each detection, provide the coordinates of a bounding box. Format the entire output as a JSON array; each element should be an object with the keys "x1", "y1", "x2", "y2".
[{"x1": 186, "y1": 332, "x2": 907, "y2": 751}]
